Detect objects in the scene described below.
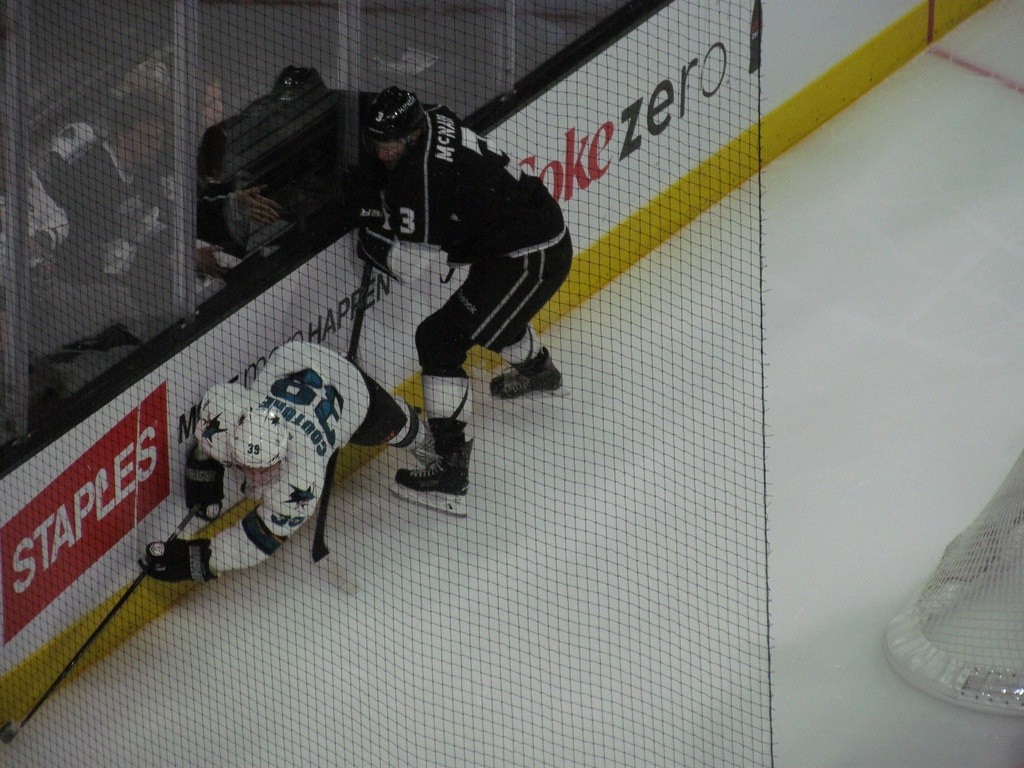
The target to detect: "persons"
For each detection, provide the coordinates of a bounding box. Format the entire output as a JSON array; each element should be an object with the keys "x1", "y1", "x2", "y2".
[
  {"x1": 136, "y1": 339, "x2": 439, "y2": 583},
  {"x1": 0, "y1": 47, "x2": 376, "y2": 396},
  {"x1": 355, "y1": 85, "x2": 573, "y2": 517},
  {"x1": 192, "y1": 64, "x2": 371, "y2": 259}
]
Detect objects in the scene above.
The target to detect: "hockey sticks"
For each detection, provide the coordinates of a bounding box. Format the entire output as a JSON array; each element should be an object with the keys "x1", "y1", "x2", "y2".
[
  {"x1": 0, "y1": 502, "x2": 202, "y2": 746},
  {"x1": 310, "y1": 259, "x2": 374, "y2": 597}
]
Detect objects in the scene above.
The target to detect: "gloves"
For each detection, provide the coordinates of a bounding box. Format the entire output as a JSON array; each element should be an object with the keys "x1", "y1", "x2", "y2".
[
  {"x1": 186, "y1": 501, "x2": 224, "y2": 520},
  {"x1": 138, "y1": 540, "x2": 217, "y2": 584}
]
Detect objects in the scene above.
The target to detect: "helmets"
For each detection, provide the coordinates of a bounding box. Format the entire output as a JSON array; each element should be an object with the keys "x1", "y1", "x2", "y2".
[
  {"x1": 231, "y1": 407, "x2": 288, "y2": 468},
  {"x1": 362, "y1": 86, "x2": 423, "y2": 142}
]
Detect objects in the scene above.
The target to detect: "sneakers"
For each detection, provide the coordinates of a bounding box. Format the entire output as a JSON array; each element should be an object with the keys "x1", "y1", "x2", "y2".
[
  {"x1": 393, "y1": 458, "x2": 470, "y2": 517},
  {"x1": 490, "y1": 347, "x2": 562, "y2": 399}
]
